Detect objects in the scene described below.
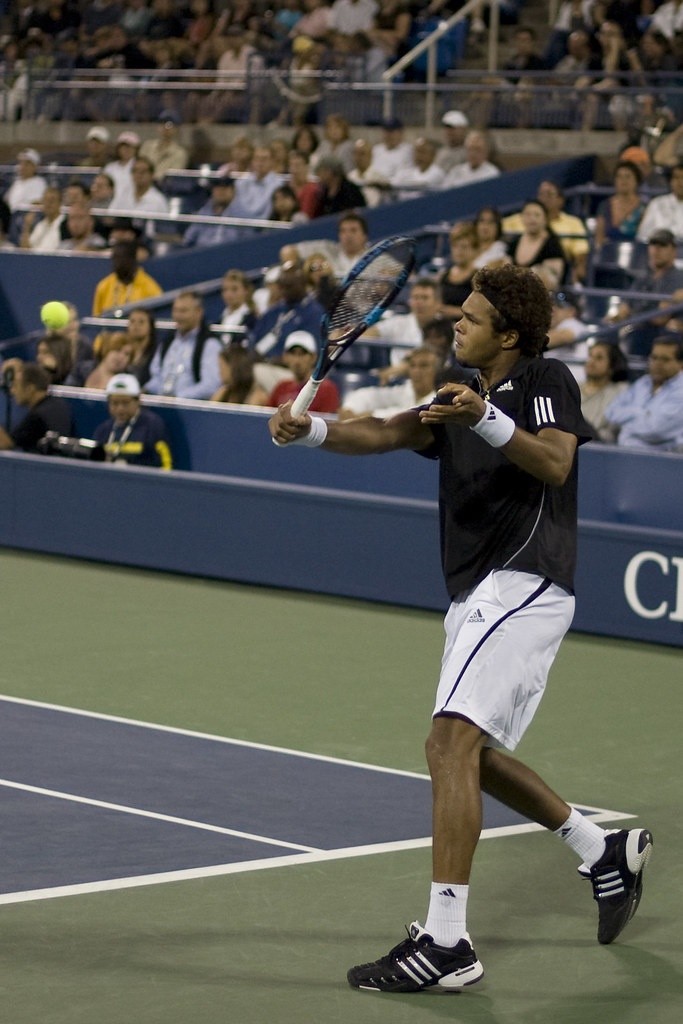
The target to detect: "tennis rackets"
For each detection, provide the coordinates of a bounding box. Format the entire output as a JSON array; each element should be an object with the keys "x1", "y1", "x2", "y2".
[{"x1": 271, "y1": 233, "x2": 420, "y2": 448}]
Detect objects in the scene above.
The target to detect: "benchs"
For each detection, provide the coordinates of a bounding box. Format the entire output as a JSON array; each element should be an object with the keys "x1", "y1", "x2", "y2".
[{"x1": 0, "y1": 165, "x2": 683, "y2": 531}]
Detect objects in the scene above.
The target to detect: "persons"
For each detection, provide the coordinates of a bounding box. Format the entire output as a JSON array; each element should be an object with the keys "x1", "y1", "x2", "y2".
[
  {"x1": 267, "y1": 267, "x2": 653, "y2": 993},
  {"x1": 0, "y1": 0, "x2": 683, "y2": 471}
]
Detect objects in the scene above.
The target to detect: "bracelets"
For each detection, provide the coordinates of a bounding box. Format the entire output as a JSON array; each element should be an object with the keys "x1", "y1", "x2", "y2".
[
  {"x1": 468, "y1": 399, "x2": 515, "y2": 447},
  {"x1": 272, "y1": 414, "x2": 328, "y2": 448}
]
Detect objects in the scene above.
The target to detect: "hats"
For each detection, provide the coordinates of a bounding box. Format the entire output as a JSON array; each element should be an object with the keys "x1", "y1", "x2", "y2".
[
  {"x1": 115, "y1": 131, "x2": 143, "y2": 148},
  {"x1": 442, "y1": 109, "x2": 468, "y2": 128},
  {"x1": 18, "y1": 146, "x2": 41, "y2": 166},
  {"x1": 85, "y1": 124, "x2": 110, "y2": 143},
  {"x1": 106, "y1": 373, "x2": 140, "y2": 400},
  {"x1": 643, "y1": 229, "x2": 677, "y2": 248},
  {"x1": 283, "y1": 329, "x2": 316, "y2": 351}
]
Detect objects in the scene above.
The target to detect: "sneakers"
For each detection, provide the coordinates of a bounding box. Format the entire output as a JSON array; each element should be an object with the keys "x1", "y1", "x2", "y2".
[
  {"x1": 576, "y1": 827, "x2": 654, "y2": 946},
  {"x1": 346, "y1": 918, "x2": 485, "y2": 995}
]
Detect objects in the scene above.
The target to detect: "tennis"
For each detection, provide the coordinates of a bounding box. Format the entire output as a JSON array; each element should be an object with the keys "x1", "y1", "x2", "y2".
[{"x1": 40, "y1": 301, "x2": 70, "y2": 330}]
